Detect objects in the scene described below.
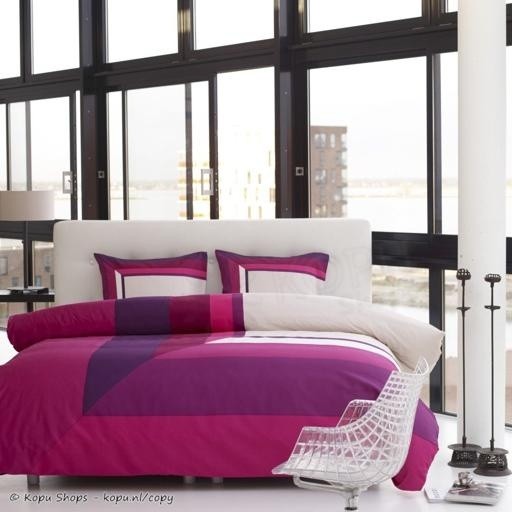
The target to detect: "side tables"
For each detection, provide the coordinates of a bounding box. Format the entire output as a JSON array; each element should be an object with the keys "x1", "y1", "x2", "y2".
[{"x1": 0, "y1": 289, "x2": 55, "y2": 313}]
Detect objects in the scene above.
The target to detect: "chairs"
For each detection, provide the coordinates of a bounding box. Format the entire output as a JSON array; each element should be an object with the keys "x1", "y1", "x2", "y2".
[{"x1": 272, "y1": 356, "x2": 430, "y2": 511}]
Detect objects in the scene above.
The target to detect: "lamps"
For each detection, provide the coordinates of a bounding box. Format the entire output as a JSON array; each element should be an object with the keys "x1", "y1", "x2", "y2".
[{"x1": 0, "y1": 191, "x2": 55, "y2": 293}]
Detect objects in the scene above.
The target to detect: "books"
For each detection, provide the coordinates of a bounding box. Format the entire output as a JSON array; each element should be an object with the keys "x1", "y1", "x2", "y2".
[
  {"x1": 444, "y1": 477, "x2": 505, "y2": 506},
  {"x1": 423, "y1": 485, "x2": 449, "y2": 504}
]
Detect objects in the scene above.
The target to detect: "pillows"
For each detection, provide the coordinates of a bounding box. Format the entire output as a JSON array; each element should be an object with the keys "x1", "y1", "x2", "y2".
[
  {"x1": 214, "y1": 250, "x2": 329, "y2": 295},
  {"x1": 93, "y1": 252, "x2": 209, "y2": 301}
]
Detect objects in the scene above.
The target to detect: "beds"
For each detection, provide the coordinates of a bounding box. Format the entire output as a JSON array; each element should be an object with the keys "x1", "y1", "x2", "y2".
[{"x1": 7, "y1": 218, "x2": 395, "y2": 487}]
